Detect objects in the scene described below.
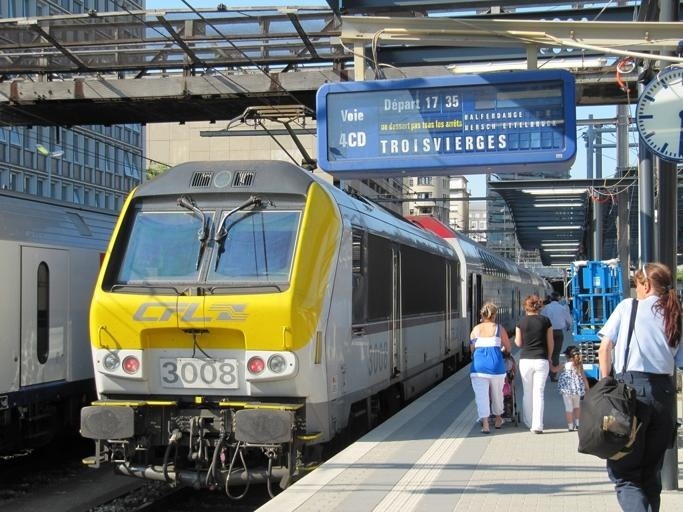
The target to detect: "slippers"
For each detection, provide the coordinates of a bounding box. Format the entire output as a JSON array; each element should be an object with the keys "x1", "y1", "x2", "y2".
[{"x1": 480, "y1": 416, "x2": 505, "y2": 433}]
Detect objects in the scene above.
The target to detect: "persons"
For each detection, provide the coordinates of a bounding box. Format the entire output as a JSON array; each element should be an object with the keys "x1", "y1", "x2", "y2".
[
  {"x1": 548, "y1": 345, "x2": 590, "y2": 432},
  {"x1": 538, "y1": 291, "x2": 572, "y2": 382},
  {"x1": 468, "y1": 302, "x2": 512, "y2": 434},
  {"x1": 596, "y1": 261, "x2": 683, "y2": 511},
  {"x1": 514, "y1": 294, "x2": 554, "y2": 434}
]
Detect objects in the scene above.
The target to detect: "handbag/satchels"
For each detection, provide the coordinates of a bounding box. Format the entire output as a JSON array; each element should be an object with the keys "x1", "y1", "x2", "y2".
[{"x1": 576, "y1": 373, "x2": 653, "y2": 464}]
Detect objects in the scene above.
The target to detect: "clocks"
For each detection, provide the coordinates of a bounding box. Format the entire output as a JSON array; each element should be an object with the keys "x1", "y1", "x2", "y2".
[{"x1": 635, "y1": 64, "x2": 683, "y2": 164}]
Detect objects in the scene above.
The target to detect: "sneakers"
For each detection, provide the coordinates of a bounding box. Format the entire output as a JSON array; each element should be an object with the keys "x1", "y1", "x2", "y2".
[{"x1": 568, "y1": 426, "x2": 579, "y2": 432}]
